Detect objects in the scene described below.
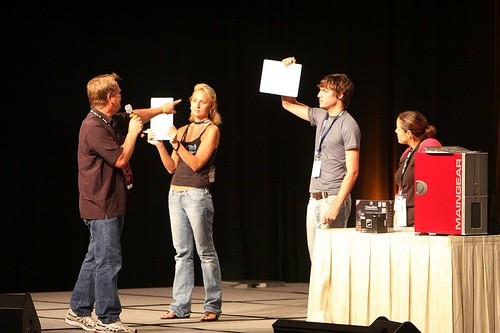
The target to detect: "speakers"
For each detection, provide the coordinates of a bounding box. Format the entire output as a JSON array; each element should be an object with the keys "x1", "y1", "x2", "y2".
[
  {"x1": 0, "y1": 291, "x2": 41, "y2": 333},
  {"x1": 272, "y1": 316, "x2": 422, "y2": 333}
]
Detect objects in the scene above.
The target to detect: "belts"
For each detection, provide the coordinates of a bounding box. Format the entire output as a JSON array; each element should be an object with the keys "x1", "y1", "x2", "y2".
[{"x1": 310, "y1": 192, "x2": 328, "y2": 200}]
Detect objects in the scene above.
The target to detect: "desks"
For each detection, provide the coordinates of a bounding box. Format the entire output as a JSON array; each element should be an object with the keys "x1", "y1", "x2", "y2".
[{"x1": 305, "y1": 226, "x2": 500, "y2": 333}]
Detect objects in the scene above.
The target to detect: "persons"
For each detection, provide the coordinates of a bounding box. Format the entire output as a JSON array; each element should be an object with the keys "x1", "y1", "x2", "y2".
[
  {"x1": 65, "y1": 73, "x2": 182, "y2": 333},
  {"x1": 395, "y1": 110, "x2": 444, "y2": 227},
  {"x1": 141, "y1": 83, "x2": 222, "y2": 322},
  {"x1": 281, "y1": 57, "x2": 360, "y2": 263}
]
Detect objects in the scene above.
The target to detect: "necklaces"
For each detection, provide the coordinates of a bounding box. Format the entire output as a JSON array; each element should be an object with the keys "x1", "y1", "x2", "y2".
[{"x1": 194, "y1": 119, "x2": 210, "y2": 124}]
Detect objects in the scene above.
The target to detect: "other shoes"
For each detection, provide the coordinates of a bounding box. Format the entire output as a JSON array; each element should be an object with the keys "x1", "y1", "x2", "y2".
[
  {"x1": 160, "y1": 311, "x2": 190, "y2": 319},
  {"x1": 200, "y1": 312, "x2": 219, "y2": 322}
]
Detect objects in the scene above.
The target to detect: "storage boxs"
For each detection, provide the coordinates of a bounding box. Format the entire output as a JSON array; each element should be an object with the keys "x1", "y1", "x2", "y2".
[
  {"x1": 355, "y1": 199, "x2": 393, "y2": 232},
  {"x1": 361, "y1": 212, "x2": 387, "y2": 232}
]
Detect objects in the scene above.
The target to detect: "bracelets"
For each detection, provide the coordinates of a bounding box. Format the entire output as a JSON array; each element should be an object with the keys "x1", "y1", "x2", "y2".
[{"x1": 162, "y1": 105, "x2": 166, "y2": 114}]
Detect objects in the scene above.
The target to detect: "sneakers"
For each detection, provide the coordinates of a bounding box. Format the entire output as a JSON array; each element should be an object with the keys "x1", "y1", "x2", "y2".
[
  {"x1": 65, "y1": 308, "x2": 96, "y2": 331},
  {"x1": 94, "y1": 319, "x2": 137, "y2": 333}
]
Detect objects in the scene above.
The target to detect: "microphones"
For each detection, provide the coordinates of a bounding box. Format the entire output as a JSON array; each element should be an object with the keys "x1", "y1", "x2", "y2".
[{"x1": 125, "y1": 104, "x2": 145, "y2": 138}]
